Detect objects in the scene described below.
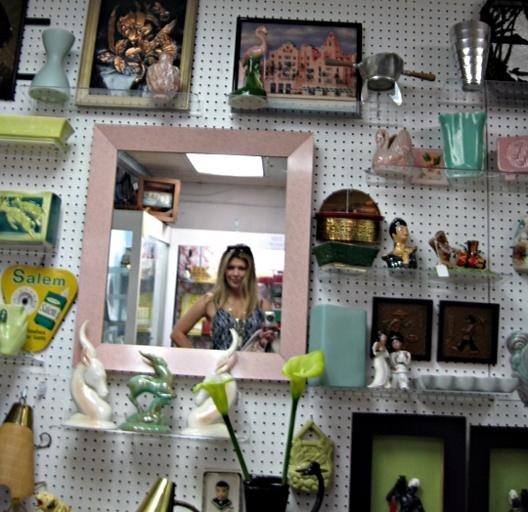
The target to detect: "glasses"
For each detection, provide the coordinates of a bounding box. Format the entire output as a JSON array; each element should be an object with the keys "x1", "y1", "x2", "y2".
[{"x1": 226, "y1": 245, "x2": 250, "y2": 252}]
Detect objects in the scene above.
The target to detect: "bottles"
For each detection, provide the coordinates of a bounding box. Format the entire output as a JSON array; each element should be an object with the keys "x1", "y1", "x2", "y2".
[{"x1": 146, "y1": 52, "x2": 182, "y2": 101}]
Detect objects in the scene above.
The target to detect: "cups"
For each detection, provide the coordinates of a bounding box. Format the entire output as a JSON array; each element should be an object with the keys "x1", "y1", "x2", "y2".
[
  {"x1": 449, "y1": 19, "x2": 493, "y2": 93},
  {"x1": 264, "y1": 310, "x2": 276, "y2": 327},
  {"x1": 0, "y1": 303, "x2": 32, "y2": 357}
]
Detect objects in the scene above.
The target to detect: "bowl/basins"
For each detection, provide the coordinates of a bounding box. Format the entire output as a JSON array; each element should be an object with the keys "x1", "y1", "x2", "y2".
[
  {"x1": 355, "y1": 53, "x2": 404, "y2": 89},
  {"x1": 313, "y1": 242, "x2": 381, "y2": 268}
]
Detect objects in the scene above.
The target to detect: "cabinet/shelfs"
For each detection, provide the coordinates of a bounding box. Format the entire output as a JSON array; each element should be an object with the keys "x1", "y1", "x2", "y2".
[
  {"x1": 101, "y1": 207, "x2": 172, "y2": 345},
  {"x1": 1, "y1": 75, "x2": 528, "y2": 445}
]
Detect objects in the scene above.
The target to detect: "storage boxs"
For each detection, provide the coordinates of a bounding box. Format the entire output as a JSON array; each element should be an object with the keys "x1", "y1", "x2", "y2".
[{"x1": 131, "y1": 174, "x2": 182, "y2": 227}]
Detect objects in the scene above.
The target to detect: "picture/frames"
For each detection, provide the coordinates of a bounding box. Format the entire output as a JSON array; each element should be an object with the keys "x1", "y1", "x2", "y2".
[
  {"x1": 229, "y1": 15, "x2": 365, "y2": 118},
  {"x1": 469, "y1": 424, "x2": 528, "y2": 512},
  {"x1": 202, "y1": 469, "x2": 243, "y2": 512},
  {"x1": 345, "y1": 410, "x2": 467, "y2": 512},
  {"x1": 440, "y1": 300, "x2": 501, "y2": 365},
  {"x1": 0, "y1": 0, "x2": 30, "y2": 100},
  {"x1": 75, "y1": 0, "x2": 200, "y2": 113},
  {"x1": 367, "y1": 292, "x2": 435, "y2": 366}
]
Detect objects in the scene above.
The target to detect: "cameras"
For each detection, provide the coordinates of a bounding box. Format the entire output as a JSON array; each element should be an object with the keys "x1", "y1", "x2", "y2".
[{"x1": 263, "y1": 311, "x2": 277, "y2": 328}]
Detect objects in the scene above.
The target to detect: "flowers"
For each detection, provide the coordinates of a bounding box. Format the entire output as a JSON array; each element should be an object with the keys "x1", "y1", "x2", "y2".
[{"x1": 192, "y1": 349, "x2": 332, "y2": 512}]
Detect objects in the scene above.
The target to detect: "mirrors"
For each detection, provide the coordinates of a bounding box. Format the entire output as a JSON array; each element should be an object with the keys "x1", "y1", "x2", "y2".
[{"x1": 67, "y1": 123, "x2": 316, "y2": 386}]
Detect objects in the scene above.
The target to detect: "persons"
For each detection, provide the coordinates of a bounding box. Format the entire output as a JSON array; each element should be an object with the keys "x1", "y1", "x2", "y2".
[
  {"x1": 208, "y1": 481, "x2": 232, "y2": 511},
  {"x1": 388, "y1": 335, "x2": 411, "y2": 391},
  {"x1": 367, "y1": 330, "x2": 393, "y2": 390},
  {"x1": 386, "y1": 476, "x2": 425, "y2": 512},
  {"x1": 381, "y1": 218, "x2": 417, "y2": 269},
  {"x1": 171, "y1": 244, "x2": 274, "y2": 353}
]
